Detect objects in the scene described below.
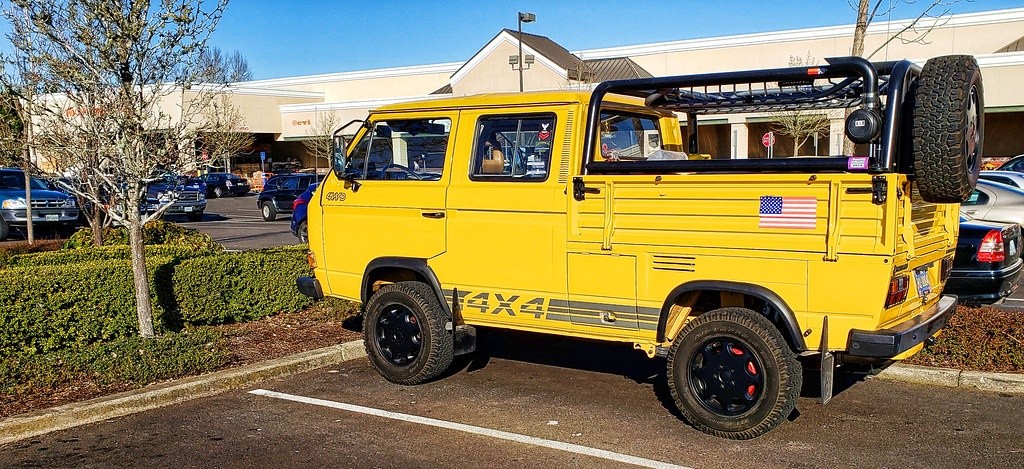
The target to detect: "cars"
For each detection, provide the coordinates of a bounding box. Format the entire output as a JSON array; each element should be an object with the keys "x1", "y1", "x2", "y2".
[
  {"x1": 198, "y1": 173, "x2": 253, "y2": 199},
  {"x1": 942, "y1": 155, "x2": 1024, "y2": 308}
]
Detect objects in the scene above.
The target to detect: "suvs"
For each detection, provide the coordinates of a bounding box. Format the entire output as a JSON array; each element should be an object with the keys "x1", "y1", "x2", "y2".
[
  {"x1": 0, "y1": 167, "x2": 207, "y2": 241},
  {"x1": 257, "y1": 174, "x2": 327, "y2": 244}
]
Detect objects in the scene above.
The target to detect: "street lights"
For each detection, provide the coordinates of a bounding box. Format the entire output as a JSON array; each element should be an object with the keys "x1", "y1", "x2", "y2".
[{"x1": 509, "y1": 12, "x2": 536, "y2": 147}]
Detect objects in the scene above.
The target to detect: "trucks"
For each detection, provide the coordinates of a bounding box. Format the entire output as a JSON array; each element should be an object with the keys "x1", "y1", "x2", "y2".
[{"x1": 297, "y1": 55, "x2": 985, "y2": 439}]
